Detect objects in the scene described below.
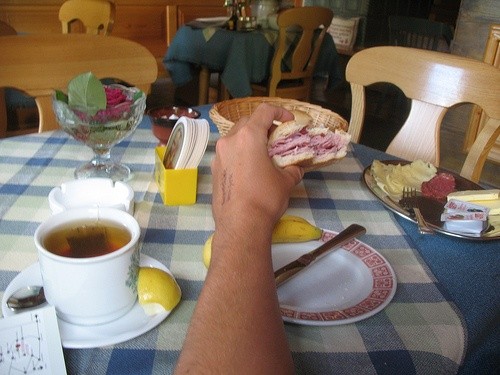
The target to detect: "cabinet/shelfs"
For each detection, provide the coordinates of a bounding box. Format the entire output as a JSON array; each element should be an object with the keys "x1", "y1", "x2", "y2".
[{"x1": 462, "y1": 25, "x2": 500, "y2": 164}]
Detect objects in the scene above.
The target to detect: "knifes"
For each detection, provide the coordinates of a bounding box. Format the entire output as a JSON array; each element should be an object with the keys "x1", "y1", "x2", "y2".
[{"x1": 274, "y1": 224, "x2": 366, "y2": 289}]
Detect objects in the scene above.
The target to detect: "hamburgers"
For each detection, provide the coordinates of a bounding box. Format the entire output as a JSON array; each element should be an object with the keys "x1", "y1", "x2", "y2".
[{"x1": 267, "y1": 120, "x2": 352, "y2": 169}]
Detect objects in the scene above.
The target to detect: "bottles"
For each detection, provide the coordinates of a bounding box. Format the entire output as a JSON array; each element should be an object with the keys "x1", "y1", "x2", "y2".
[
  {"x1": 229, "y1": 7, "x2": 237, "y2": 31},
  {"x1": 244, "y1": 17, "x2": 251, "y2": 32},
  {"x1": 241, "y1": 0, "x2": 246, "y2": 17},
  {"x1": 251, "y1": 16, "x2": 257, "y2": 31},
  {"x1": 238, "y1": 17, "x2": 244, "y2": 31}
]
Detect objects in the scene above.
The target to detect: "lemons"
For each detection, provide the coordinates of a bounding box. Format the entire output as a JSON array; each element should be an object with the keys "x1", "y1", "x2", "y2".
[{"x1": 138, "y1": 267, "x2": 182, "y2": 315}]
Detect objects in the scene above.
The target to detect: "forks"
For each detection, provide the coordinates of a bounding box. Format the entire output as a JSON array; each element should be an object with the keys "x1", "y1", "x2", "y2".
[{"x1": 403, "y1": 186, "x2": 433, "y2": 234}]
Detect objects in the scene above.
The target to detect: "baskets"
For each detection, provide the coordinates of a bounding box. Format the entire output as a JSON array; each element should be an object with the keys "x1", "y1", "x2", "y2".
[{"x1": 209, "y1": 96, "x2": 349, "y2": 135}]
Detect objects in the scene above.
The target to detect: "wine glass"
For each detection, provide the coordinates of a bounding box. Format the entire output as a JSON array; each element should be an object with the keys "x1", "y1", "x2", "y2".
[
  {"x1": 147, "y1": 105, "x2": 201, "y2": 146},
  {"x1": 50, "y1": 84, "x2": 146, "y2": 183}
]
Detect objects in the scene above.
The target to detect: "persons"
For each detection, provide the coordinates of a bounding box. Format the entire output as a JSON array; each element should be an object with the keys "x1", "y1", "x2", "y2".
[{"x1": 174, "y1": 102, "x2": 305, "y2": 375}]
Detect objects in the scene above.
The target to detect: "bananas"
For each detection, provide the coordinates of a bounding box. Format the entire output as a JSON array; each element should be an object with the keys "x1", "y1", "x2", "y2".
[{"x1": 202, "y1": 216, "x2": 324, "y2": 270}]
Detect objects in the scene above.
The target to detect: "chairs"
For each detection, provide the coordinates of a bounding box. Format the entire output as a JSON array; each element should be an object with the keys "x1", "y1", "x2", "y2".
[
  {"x1": 0, "y1": 34, "x2": 158, "y2": 133},
  {"x1": 250, "y1": 6, "x2": 334, "y2": 103},
  {"x1": 345, "y1": 46, "x2": 500, "y2": 183},
  {"x1": 58, "y1": 0, "x2": 116, "y2": 35}
]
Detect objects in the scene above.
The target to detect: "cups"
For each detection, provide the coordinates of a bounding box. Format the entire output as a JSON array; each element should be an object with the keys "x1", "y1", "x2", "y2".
[{"x1": 34, "y1": 206, "x2": 141, "y2": 326}]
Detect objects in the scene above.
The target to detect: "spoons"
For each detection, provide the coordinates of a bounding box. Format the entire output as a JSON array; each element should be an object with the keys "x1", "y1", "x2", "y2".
[{"x1": 6, "y1": 286, "x2": 46, "y2": 313}]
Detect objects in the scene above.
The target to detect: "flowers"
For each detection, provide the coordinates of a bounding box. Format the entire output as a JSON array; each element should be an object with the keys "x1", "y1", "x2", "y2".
[{"x1": 54, "y1": 71, "x2": 143, "y2": 132}]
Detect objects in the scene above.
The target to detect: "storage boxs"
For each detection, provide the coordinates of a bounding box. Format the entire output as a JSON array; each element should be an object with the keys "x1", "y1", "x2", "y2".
[{"x1": 155, "y1": 146, "x2": 198, "y2": 205}]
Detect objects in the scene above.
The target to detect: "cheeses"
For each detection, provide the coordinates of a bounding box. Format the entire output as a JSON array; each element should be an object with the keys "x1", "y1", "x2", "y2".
[
  {"x1": 446, "y1": 188, "x2": 500, "y2": 216},
  {"x1": 373, "y1": 159, "x2": 437, "y2": 193}
]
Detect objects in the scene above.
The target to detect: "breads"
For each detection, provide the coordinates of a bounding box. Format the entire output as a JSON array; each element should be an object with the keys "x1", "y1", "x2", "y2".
[{"x1": 288, "y1": 109, "x2": 312, "y2": 122}]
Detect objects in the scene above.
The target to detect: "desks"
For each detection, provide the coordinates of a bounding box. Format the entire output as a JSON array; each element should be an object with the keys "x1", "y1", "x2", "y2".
[
  {"x1": 0, "y1": 105, "x2": 500, "y2": 375},
  {"x1": 162, "y1": 21, "x2": 343, "y2": 106}
]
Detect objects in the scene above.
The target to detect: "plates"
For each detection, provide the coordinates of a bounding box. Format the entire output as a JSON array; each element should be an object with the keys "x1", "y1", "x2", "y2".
[
  {"x1": 1, "y1": 253, "x2": 176, "y2": 348},
  {"x1": 203, "y1": 228, "x2": 397, "y2": 326},
  {"x1": 363, "y1": 160, "x2": 500, "y2": 241}
]
